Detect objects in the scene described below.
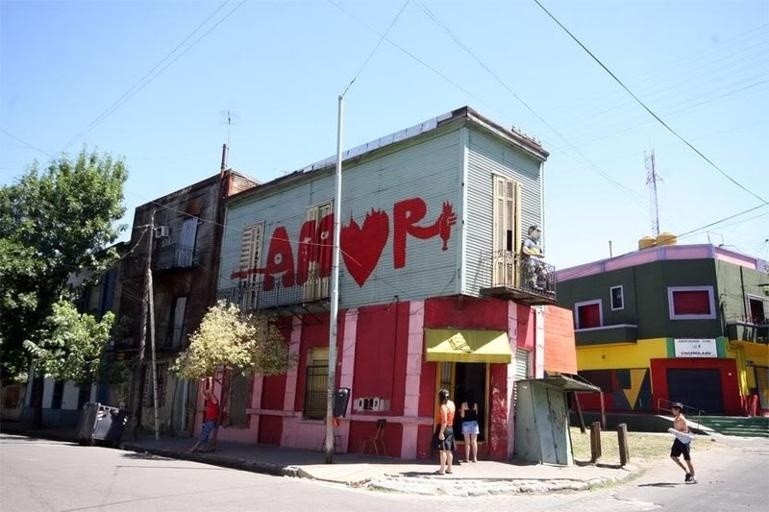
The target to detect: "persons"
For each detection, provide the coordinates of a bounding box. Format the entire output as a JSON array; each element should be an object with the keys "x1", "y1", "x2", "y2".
[
  {"x1": 458, "y1": 390, "x2": 481, "y2": 464},
  {"x1": 522, "y1": 224, "x2": 548, "y2": 291},
  {"x1": 187, "y1": 386, "x2": 220, "y2": 454},
  {"x1": 430, "y1": 388, "x2": 457, "y2": 475},
  {"x1": 667, "y1": 402, "x2": 697, "y2": 484}
]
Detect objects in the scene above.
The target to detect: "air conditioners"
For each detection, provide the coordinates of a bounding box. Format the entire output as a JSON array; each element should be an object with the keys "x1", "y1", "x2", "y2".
[{"x1": 155, "y1": 226, "x2": 169, "y2": 239}]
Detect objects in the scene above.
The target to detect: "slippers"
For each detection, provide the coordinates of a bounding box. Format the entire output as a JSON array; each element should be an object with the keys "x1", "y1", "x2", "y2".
[
  {"x1": 433, "y1": 471, "x2": 445, "y2": 475},
  {"x1": 446, "y1": 471, "x2": 453, "y2": 474}
]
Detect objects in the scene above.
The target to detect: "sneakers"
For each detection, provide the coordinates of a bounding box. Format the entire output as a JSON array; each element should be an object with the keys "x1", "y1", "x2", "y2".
[
  {"x1": 686, "y1": 478, "x2": 696, "y2": 484},
  {"x1": 685, "y1": 473, "x2": 690, "y2": 482}
]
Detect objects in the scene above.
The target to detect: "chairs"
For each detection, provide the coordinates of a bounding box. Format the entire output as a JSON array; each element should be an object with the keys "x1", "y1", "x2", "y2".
[
  {"x1": 321, "y1": 416, "x2": 344, "y2": 457},
  {"x1": 357, "y1": 418, "x2": 387, "y2": 457}
]
